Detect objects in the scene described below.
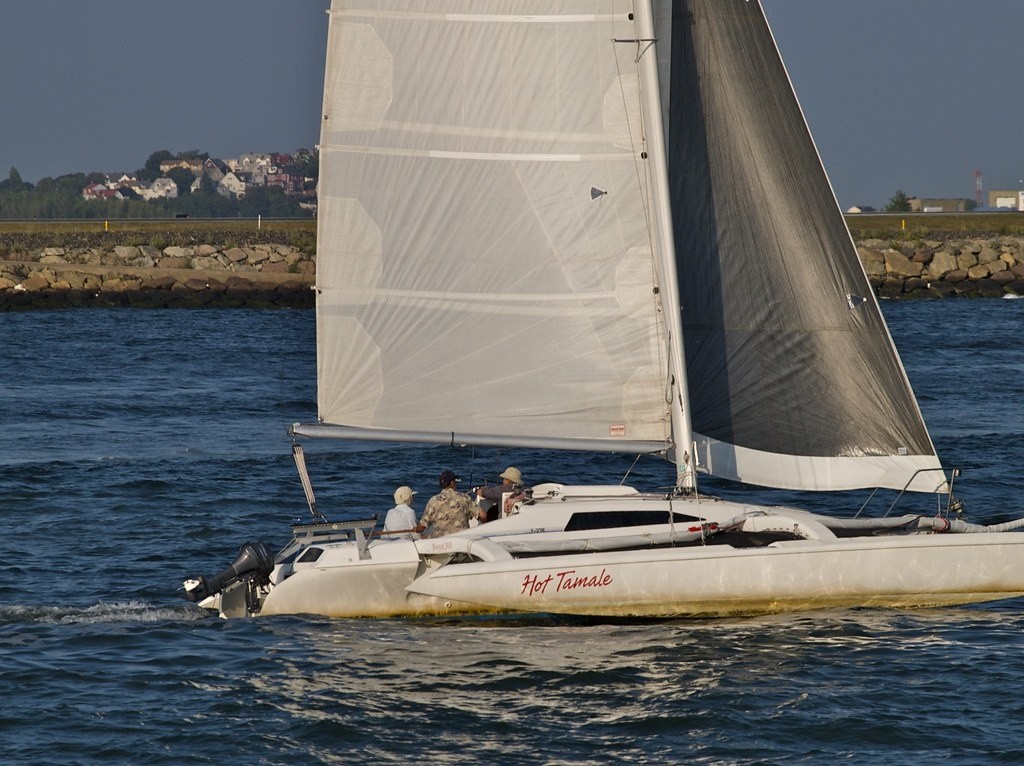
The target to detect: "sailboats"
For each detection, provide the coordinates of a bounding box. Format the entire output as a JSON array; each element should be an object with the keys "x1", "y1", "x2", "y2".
[{"x1": 183, "y1": 2, "x2": 1024, "y2": 624}]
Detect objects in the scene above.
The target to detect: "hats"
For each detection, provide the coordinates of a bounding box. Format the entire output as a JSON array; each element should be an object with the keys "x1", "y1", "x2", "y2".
[
  {"x1": 499, "y1": 467, "x2": 522, "y2": 484},
  {"x1": 440, "y1": 471, "x2": 460, "y2": 481},
  {"x1": 394, "y1": 486, "x2": 419, "y2": 505}
]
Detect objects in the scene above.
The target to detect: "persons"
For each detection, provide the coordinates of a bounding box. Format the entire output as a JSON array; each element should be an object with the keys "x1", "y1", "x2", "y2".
[
  {"x1": 413, "y1": 469, "x2": 486, "y2": 539},
  {"x1": 380, "y1": 486, "x2": 422, "y2": 540},
  {"x1": 476, "y1": 466, "x2": 523, "y2": 524}
]
